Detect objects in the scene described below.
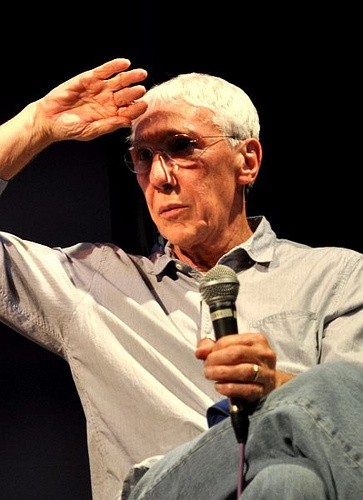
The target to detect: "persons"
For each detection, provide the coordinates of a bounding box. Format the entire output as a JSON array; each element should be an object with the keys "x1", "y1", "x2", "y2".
[{"x1": 0, "y1": 58, "x2": 363, "y2": 500}]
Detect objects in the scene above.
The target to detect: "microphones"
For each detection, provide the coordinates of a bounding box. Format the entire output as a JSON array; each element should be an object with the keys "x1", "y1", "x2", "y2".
[{"x1": 199, "y1": 265, "x2": 249, "y2": 443}]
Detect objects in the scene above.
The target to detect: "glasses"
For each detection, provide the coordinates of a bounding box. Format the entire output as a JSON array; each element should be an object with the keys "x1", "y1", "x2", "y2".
[{"x1": 124, "y1": 133, "x2": 235, "y2": 174}]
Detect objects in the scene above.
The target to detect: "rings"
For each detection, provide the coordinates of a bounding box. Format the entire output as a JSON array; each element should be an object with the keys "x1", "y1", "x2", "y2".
[{"x1": 247, "y1": 364, "x2": 259, "y2": 383}]
